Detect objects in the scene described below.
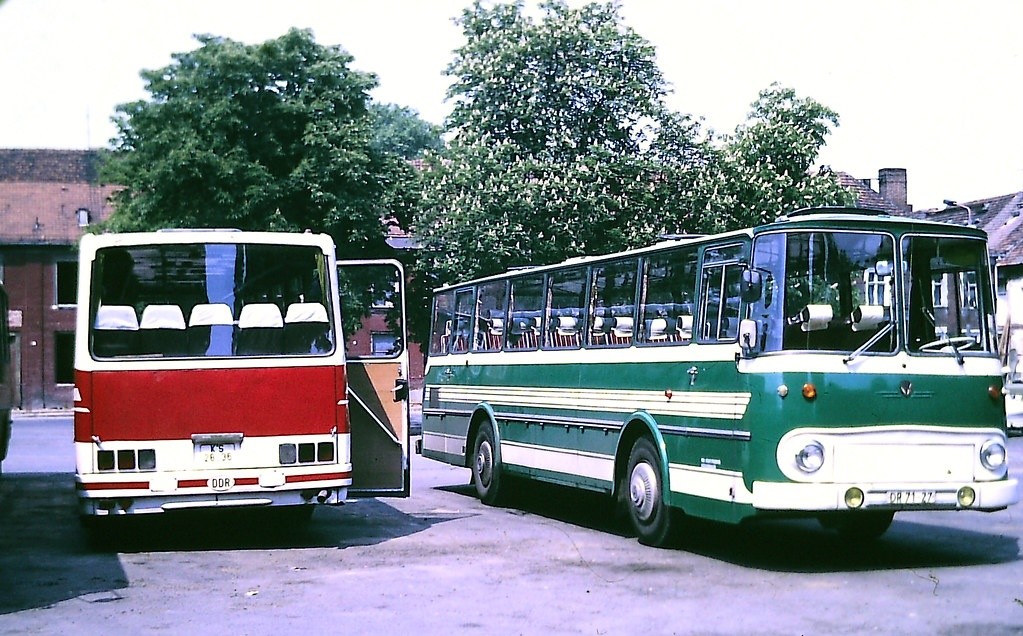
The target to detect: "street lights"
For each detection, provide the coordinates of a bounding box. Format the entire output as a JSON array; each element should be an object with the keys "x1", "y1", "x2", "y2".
[{"x1": 942, "y1": 199, "x2": 973, "y2": 226}]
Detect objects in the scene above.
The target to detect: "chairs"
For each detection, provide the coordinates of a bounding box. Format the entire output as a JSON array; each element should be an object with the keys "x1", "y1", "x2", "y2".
[
  {"x1": 434, "y1": 300, "x2": 742, "y2": 356},
  {"x1": 88, "y1": 302, "x2": 331, "y2": 363}
]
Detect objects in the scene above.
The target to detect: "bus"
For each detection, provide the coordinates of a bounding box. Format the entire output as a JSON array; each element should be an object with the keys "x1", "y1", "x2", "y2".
[
  {"x1": 74, "y1": 225, "x2": 411, "y2": 516},
  {"x1": 0, "y1": 280, "x2": 19, "y2": 461},
  {"x1": 415, "y1": 202, "x2": 1018, "y2": 547}
]
